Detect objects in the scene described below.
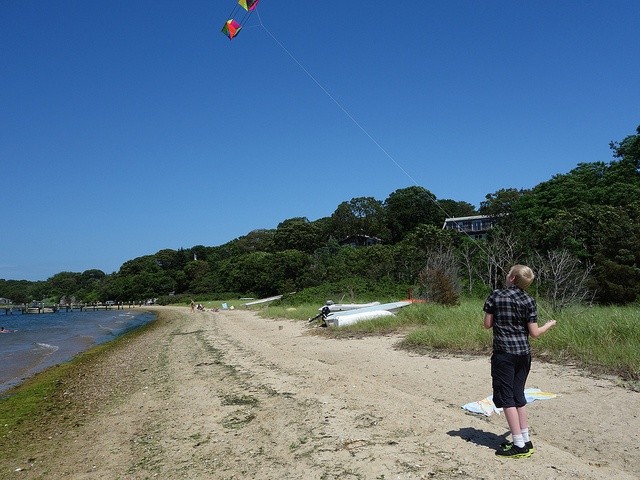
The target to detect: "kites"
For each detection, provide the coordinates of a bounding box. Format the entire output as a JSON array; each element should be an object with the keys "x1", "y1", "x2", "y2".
[{"x1": 220, "y1": 0, "x2": 258, "y2": 40}]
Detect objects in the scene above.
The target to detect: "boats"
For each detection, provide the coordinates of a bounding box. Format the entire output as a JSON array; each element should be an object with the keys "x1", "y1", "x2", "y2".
[
  {"x1": 334, "y1": 310, "x2": 396, "y2": 327},
  {"x1": 318, "y1": 301, "x2": 381, "y2": 310},
  {"x1": 307, "y1": 300, "x2": 413, "y2": 326}
]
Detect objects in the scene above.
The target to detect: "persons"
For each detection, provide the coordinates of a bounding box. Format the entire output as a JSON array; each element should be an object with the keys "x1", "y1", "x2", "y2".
[{"x1": 482, "y1": 261, "x2": 558, "y2": 461}]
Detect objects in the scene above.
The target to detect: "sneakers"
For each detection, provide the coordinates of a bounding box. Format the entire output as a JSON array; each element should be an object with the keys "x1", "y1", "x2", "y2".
[
  {"x1": 499, "y1": 441, "x2": 534, "y2": 454},
  {"x1": 495, "y1": 441, "x2": 532, "y2": 459}
]
